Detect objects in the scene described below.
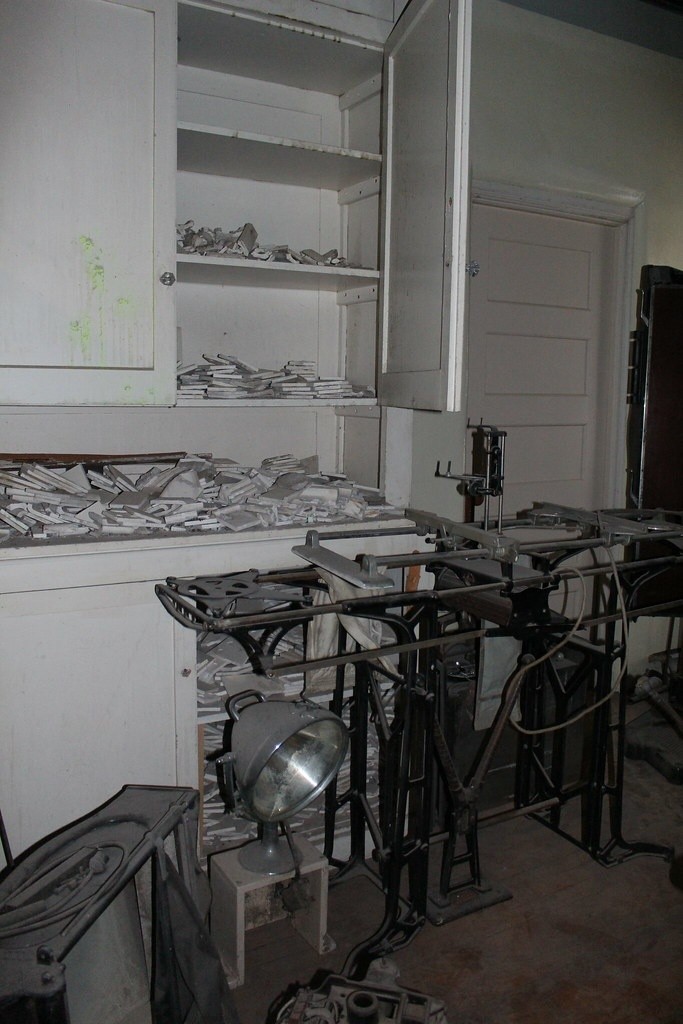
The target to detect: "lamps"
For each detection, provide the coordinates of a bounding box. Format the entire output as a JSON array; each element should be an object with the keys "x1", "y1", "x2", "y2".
[{"x1": 217, "y1": 689, "x2": 349, "y2": 872}]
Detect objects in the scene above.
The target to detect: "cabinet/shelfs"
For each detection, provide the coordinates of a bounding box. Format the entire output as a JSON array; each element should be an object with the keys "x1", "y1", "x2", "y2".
[
  {"x1": 0, "y1": 505, "x2": 446, "y2": 872},
  {"x1": 0, "y1": 0, "x2": 474, "y2": 417}
]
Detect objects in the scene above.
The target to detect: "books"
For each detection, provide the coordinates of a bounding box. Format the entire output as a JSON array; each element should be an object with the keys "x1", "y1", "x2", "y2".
[
  {"x1": 195, "y1": 624, "x2": 396, "y2": 846},
  {"x1": 175, "y1": 219, "x2": 348, "y2": 267},
  {"x1": 176, "y1": 354, "x2": 375, "y2": 400},
  {"x1": 0, "y1": 453, "x2": 395, "y2": 541}
]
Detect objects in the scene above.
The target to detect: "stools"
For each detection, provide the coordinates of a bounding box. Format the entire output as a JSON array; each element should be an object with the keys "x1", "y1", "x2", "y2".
[{"x1": 212, "y1": 832, "x2": 334, "y2": 989}]
